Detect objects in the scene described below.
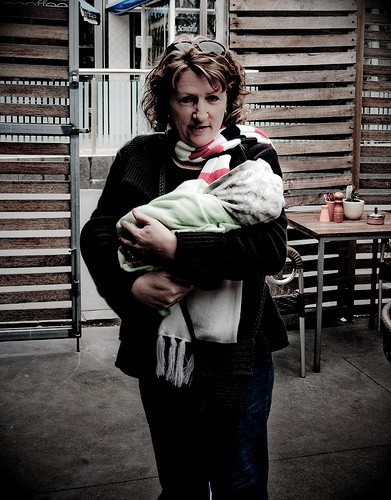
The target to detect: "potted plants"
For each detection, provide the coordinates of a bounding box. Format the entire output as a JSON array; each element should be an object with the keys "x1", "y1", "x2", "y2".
[{"x1": 342, "y1": 185, "x2": 365, "y2": 221}]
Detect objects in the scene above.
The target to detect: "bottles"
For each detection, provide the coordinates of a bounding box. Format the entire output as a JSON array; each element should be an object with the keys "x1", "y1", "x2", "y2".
[
  {"x1": 333, "y1": 200, "x2": 344, "y2": 223},
  {"x1": 319, "y1": 205, "x2": 329, "y2": 222}
]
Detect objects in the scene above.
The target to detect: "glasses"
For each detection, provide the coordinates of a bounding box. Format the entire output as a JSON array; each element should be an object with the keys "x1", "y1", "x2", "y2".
[{"x1": 161, "y1": 40, "x2": 230, "y2": 65}]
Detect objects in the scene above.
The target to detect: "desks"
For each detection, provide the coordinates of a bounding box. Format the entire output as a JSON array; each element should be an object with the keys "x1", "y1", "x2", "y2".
[{"x1": 287, "y1": 211, "x2": 391, "y2": 373}]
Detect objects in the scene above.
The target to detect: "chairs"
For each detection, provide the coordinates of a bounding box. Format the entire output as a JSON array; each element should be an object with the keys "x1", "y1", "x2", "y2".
[
  {"x1": 378, "y1": 239, "x2": 391, "y2": 331},
  {"x1": 266, "y1": 247, "x2": 308, "y2": 379}
]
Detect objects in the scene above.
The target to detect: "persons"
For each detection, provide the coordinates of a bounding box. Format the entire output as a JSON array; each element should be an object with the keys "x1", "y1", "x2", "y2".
[
  {"x1": 116, "y1": 159, "x2": 285, "y2": 233},
  {"x1": 80, "y1": 33, "x2": 287, "y2": 499}
]
